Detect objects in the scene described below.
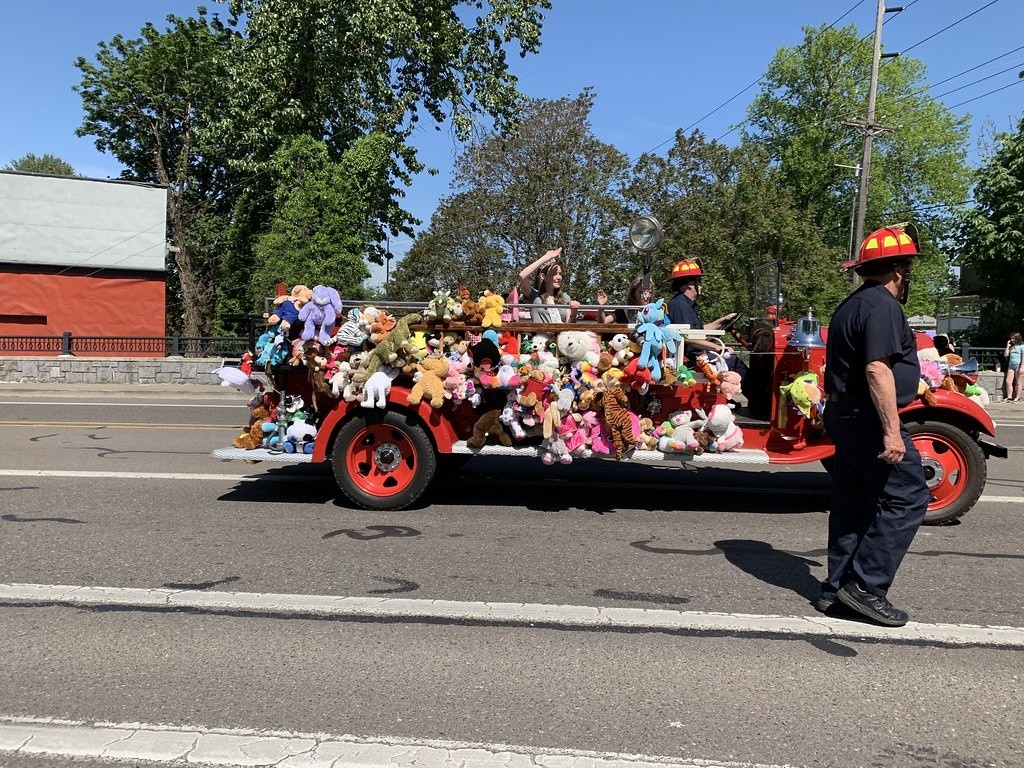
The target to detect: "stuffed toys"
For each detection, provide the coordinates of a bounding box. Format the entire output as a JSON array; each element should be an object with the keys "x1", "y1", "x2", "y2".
[
  {"x1": 916, "y1": 348, "x2": 989, "y2": 409},
  {"x1": 232, "y1": 285, "x2": 745, "y2": 465},
  {"x1": 779, "y1": 371, "x2": 820, "y2": 419}
]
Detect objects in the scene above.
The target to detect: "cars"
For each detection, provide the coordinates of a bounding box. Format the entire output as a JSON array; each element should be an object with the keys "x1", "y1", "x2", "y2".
[{"x1": 239, "y1": 257, "x2": 1009, "y2": 526}]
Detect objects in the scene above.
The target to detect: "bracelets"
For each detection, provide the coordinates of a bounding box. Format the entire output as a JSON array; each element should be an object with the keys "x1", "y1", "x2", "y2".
[{"x1": 569, "y1": 314, "x2": 578, "y2": 320}]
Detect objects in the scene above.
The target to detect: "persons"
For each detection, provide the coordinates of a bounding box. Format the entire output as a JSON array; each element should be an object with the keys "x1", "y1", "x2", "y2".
[
  {"x1": 518, "y1": 247, "x2": 580, "y2": 323},
  {"x1": 663, "y1": 260, "x2": 749, "y2": 377},
  {"x1": 598, "y1": 276, "x2": 655, "y2": 323},
  {"x1": 816, "y1": 224, "x2": 931, "y2": 625},
  {"x1": 1003, "y1": 333, "x2": 1024, "y2": 403}
]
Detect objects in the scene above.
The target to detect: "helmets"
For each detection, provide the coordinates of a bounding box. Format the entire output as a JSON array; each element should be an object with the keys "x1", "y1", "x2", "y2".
[
  {"x1": 663, "y1": 257, "x2": 704, "y2": 283},
  {"x1": 840, "y1": 222, "x2": 924, "y2": 271}
]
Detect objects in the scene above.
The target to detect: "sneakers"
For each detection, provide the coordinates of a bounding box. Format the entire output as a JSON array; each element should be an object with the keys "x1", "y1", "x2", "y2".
[
  {"x1": 837, "y1": 577, "x2": 909, "y2": 625},
  {"x1": 817, "y1": 589, "x2": 871, "y2": 620}
]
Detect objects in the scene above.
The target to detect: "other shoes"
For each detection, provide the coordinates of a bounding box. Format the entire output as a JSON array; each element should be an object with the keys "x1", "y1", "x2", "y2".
[
  {"x1": 1012, "y1": 397, "x2": 1023, "y2": 404},
  {"x1": 1000, "y1": 398, "x2": 1012, "y2": 404}
]
